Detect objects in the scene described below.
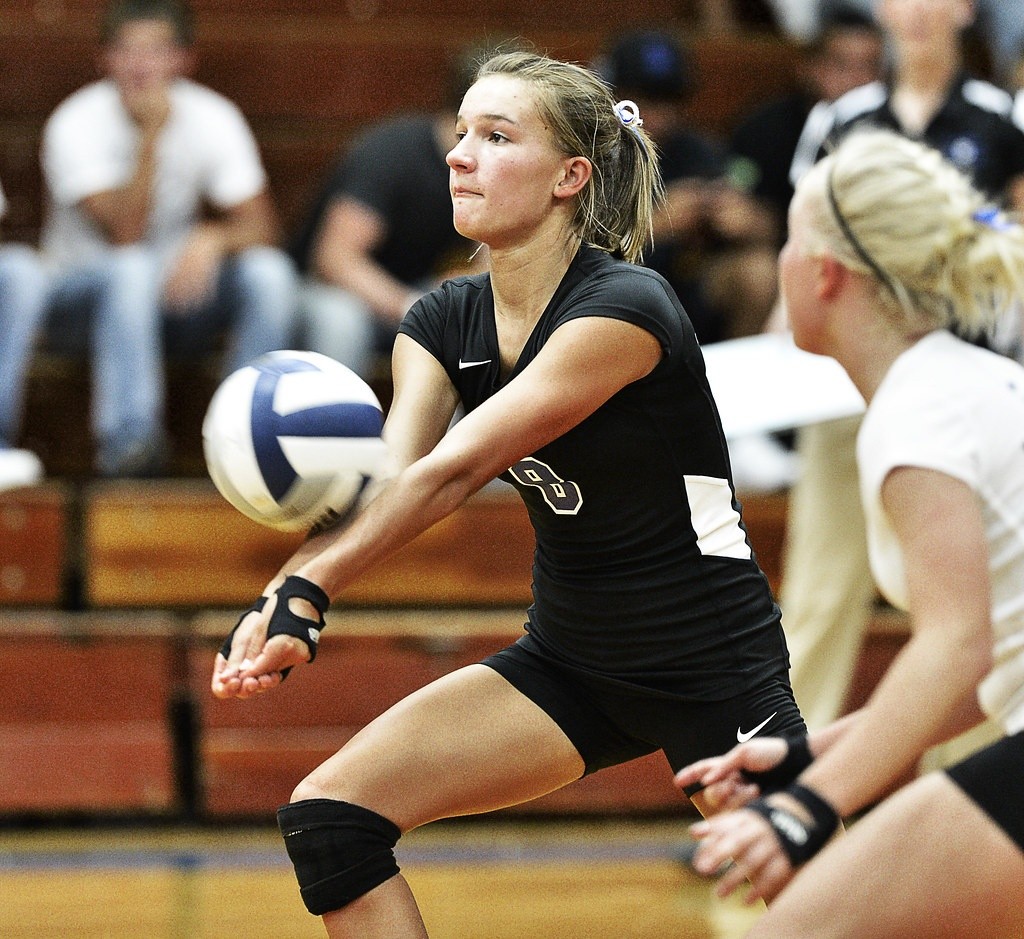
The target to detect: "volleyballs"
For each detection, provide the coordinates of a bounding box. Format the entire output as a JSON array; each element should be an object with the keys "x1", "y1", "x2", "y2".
[{"x1": 200, "y1": 347, "x2": 389, "y2": 534}]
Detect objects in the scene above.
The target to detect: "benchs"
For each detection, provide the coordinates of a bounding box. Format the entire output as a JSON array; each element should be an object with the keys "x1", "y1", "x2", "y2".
[{"x1": 0, "y1": 2, "x2": 989, "y2": 824}]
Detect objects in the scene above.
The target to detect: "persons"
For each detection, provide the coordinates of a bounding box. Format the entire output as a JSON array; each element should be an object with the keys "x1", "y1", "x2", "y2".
[
  {"x1": 211, "y1": 47, "x2": 818, "y2": 939},
  {"x1": 34, "y1": 0, "x2": 304, "y2": 485},
  {"x1": 314, "y1": 0, "x2": 1024, "y2": 407},
  {"x1": 670, "y1": 125, "x2": 1024, "y2": 939}
]
